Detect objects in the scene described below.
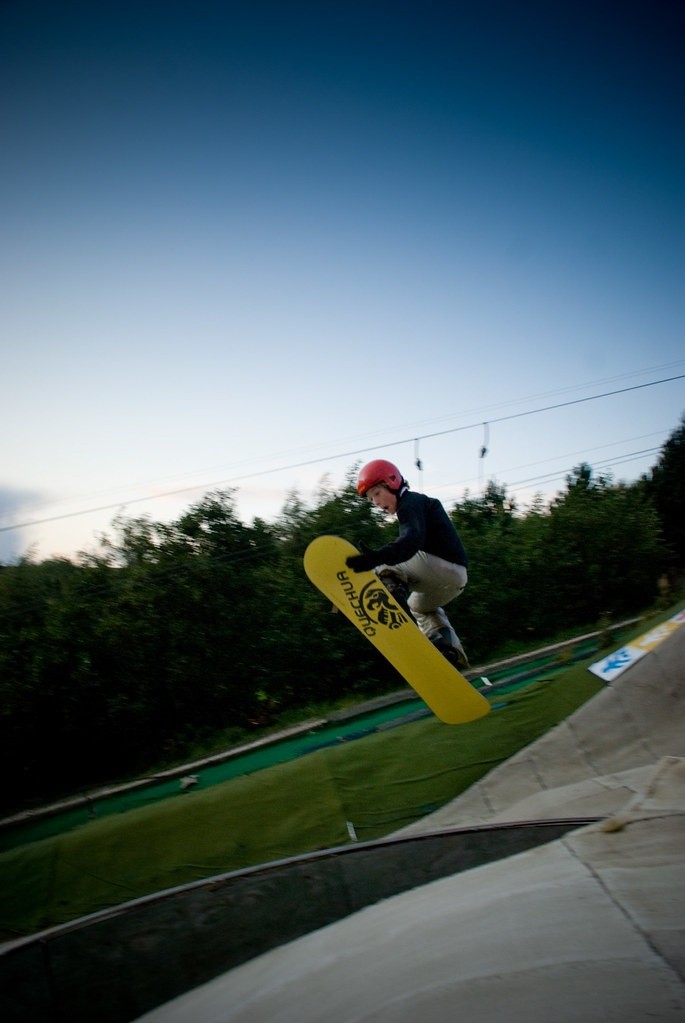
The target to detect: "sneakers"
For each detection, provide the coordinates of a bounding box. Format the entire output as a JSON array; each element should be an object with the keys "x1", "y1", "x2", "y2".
[
  {"x1": 385, "y1": 582, "x2": 406, "y2": 603},
  {"x1": 438, "y1": 645, "x2": 462, "y2": 672}
]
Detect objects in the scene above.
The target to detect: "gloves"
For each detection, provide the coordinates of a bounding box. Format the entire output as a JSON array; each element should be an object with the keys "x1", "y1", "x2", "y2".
[{"x1": 346, "y1": 540, "x2": 388, "y2": 573}]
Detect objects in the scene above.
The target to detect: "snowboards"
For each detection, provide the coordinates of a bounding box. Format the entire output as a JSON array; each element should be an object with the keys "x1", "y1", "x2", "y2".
[{"x1": 303, "y1": 535, "x2": 491, "y2": 725}]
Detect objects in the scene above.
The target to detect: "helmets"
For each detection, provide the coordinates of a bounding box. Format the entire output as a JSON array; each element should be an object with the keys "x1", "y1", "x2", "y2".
[{"x1": 356, "y1": 460, "x2": 404, "y2": 497}]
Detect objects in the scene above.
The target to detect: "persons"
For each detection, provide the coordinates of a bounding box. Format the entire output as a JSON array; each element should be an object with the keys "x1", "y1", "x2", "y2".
[
  {"x1": 657, "y1": 573, "x2": 672, "y2": 597},
  {"x1": 345, "y1": 460, "x2": 468, "y2": 672}
]
